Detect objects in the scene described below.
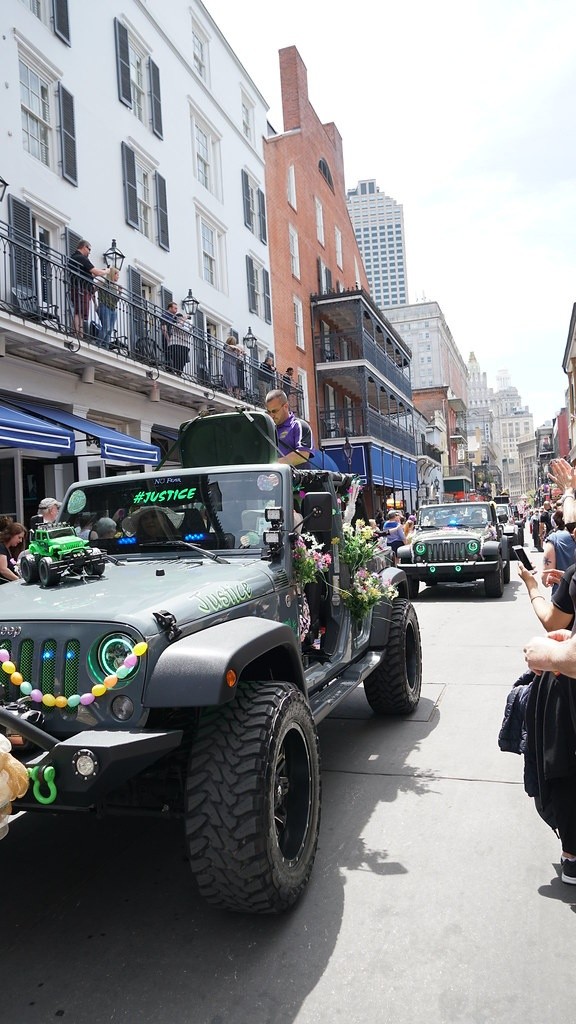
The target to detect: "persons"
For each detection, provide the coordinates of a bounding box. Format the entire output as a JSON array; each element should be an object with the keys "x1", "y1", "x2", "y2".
[
  {"x1": 368, "y1": 506, "x2": 417, "y2": 570},
  {"x1": 257, "y1": 357, "x2": 276, "y2": 407},
  {"x1": 283, "y1": 367, "x2": 294, "y2": 397},
  {"x1": 265, "y1": 390, "x2": 316, "y2": 465},
  {"x1": 242, "y1": 497, "x2": 311, "y2": 641},
  {"x1": 471, "y1": 510, "x2": 483, "y2": 523},
  {"x1": 160, "y1": 302, "x2": 193, "y2": 376},
  {"x1": 0, "y1": 497, "x2": 171, "y2": 587},
  {"x1": 518, "y1": 458, "x2": 576, "y2": 886},
  {"x1": 91, "y1": 267, "x2": 122, "y2": 349},
  {"x1": 221, "y1": 336, "x2": 248, "y2": 399},
  {"x1": 67, "y1": 240, "x2": 110, "y2": 340}
]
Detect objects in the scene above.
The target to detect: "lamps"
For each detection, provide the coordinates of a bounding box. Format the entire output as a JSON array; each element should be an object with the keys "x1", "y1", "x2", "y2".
[
  {"x1": 0, "y1": 175, "x2": 9, "y2": 201},
  {"x1": 243, "y1": 326, "x2": 257, "y2": 348},
  {"x1": 102, "y1": 237, "x2": 125, "y2": 271},
  {"x1": 182, "y1": 289, "x2": 199, "y2": 314}
]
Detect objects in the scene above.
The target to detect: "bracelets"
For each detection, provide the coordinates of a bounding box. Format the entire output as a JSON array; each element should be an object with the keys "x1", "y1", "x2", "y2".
[
  {"x1": 563, "y1": 495, "x2": 574, "y2": 502},
  {"x1": 531, "y1": 596, "x2": 545, "y2": 603}
]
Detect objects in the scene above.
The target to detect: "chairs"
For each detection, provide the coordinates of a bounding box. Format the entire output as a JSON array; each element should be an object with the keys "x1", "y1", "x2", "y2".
[
  {"x1": 323, "y1": 347, "x2": 336, "y2": 361},
  {"x1": 322, "y1": 419, "x2": 340, "y2": 437}
]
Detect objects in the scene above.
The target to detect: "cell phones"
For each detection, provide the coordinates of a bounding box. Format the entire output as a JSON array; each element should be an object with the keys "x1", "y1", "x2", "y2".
[{"x1": 512, "y1": 545, "x2": 535, "y2": 575}]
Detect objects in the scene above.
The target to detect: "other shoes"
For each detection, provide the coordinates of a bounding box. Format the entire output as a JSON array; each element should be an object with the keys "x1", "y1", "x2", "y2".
[{"x1": 560, "y1": 857, "x2": 576, "y2": 883}]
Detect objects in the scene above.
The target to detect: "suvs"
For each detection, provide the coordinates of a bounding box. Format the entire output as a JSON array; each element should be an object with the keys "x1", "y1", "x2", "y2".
[
  {"x1": 396, "y1": 500, "x2": 526, "y2": 597},
  {"x1": 1, "y1": 462, "x2": 423, "y2": 925}
]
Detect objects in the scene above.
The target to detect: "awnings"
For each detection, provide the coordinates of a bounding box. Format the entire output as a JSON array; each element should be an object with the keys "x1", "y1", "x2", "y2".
[
  {"x1": 0, "y1": 404, "x2": 75, "y2": 453},
  {"x1": 0, "y1": 395, "x2": 161, "y2": 464},
  {"x1": 151, "y1": 430, "x2": 178, "y2": 453}
]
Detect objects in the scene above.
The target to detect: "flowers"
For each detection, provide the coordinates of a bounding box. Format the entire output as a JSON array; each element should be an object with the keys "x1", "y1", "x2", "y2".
[
  {"x1": 336, "y1": 570, "x2": 399, "y2": 647},
  {"x1": 331, "y1": 520, "x2": 390, "y2": 577},
  {"x1": 289, "y1": 534, "x2": 332, "y2": 592}
]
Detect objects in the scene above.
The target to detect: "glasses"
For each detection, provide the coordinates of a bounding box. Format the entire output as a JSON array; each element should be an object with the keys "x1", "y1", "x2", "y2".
[
  {"x1": 265, "y1": 404, "x2": 286, "y2": 415},
  {"x1": 85, "y1": 245, "x2": 91, "y2": 252}
]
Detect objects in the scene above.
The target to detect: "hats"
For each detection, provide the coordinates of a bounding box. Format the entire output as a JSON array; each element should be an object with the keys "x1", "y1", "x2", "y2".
[
  {"x1": 387, "y1": 511, "x2": 398, "y2": 517},
  {"x1": 38, "y1": 498, "x2": 63, "y2": 511},
  {"x1": 408, "y1": 515, "x2": 417, "y2": 523},
  {"x1": 286, "y1": 368, "x2": 292, "y2": 372}
]
